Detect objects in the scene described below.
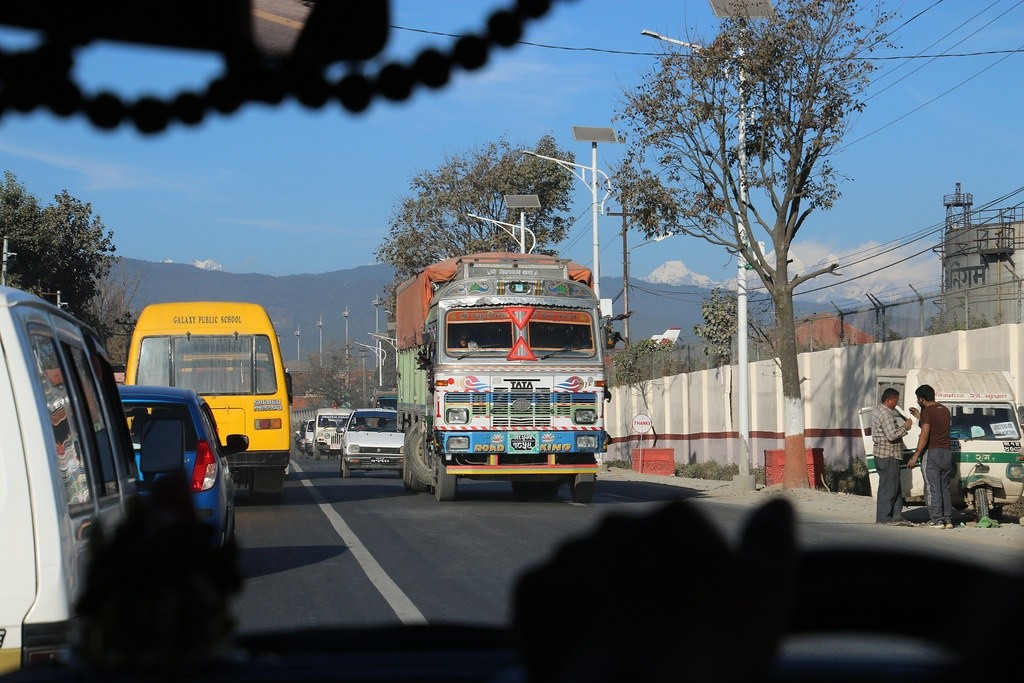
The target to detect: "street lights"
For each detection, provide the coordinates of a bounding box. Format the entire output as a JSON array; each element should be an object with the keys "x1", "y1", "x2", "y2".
[
  {"x1": 642, "y1": 28, "x2": 745, "y2": 475},
  {"x1": 522, "y1": 149, "x2": 612, "y2": 301},
  {"x1": 354, "y1": 342, "x2": 385, "y2": 388},
  {"x1": 1, "y1": 252, "x2": 17, "y2": 286},
  {"x1": 623, "y1": 229, "x2": 674, "y2": 349},
  {"x1": 294, "y1": 298, "x2": 383, "y2": 371},
  {"x1": 368, "y1": 333, "x2": 400, "y2": 379},
  {"x1": 57, "y1": 302, "x2": 75, "y2": 315},
  {"x1": 468, "y1": 211, "x2": 536, "y2": 255}
]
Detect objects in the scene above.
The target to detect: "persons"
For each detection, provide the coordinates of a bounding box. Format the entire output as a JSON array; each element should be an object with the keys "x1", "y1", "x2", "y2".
[
  {"x1": 872, "y1": 387, "x2": 913, "y2": 527},
  {"x1": 907, "y1": 384, "x2": 954, "y2": 528},
  {"x1": 508, "y1": 495, "x2": 796, "y2": 683},
  {"x1": 457, "y1": 330, "x2": 470, "y2": 347}
]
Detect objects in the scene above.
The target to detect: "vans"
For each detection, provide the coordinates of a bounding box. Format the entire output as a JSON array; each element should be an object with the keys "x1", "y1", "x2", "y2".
[
  {"x1": 305, "y1": 420, "x2": 338, "y2": 456},
  {"x1": 0, "y1": 284, "x2": 181, "y2": 683}
]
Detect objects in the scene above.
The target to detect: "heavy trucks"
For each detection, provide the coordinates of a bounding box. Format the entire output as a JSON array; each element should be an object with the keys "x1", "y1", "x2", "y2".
[{"x1": 394, "y1": 249, "x2": 612, "y2": 503}]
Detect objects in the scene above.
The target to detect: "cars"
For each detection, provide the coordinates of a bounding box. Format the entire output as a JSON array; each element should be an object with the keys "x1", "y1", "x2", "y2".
[
  {"x1": 115, "y1": 383, "x2": 250, "y2": 575},
  {"x1": 337, "y1": 408, "x2": 406, "y2": 478},
  {"x1": 296, "y1": 423, "x2": 306, "y2": 454}
]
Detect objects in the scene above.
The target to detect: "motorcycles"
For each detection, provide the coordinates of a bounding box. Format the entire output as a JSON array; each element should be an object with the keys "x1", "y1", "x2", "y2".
[{"x1": 858, "y1": 366, "x2": 1024, "y2": 526}]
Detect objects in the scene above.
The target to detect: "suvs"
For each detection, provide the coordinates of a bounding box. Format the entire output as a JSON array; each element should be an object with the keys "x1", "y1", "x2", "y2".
[{"x1": 311, "y1": 408, "x2": 356, "y2": 460}]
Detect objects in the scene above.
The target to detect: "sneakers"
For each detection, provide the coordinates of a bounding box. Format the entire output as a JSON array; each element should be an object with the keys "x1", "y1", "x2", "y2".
[
  {"x1": 945, "y1": 520, "x2": 954, "y2": 528},
  {"x1": 926, "y1": 520, "x2": 944, "y2": 529}
]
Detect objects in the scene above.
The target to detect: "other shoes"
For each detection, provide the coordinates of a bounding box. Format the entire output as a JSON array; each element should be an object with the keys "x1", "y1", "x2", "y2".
[{"x1": 893, "y1": 518, "x2": 913, "y2": 527}]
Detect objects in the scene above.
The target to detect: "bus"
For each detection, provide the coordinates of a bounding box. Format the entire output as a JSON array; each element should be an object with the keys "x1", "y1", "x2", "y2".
[{"x1": 124, "y1": 300, "x2": 294, "y2": 505}]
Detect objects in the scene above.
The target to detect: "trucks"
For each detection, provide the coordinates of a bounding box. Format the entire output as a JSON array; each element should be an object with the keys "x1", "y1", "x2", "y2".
[{"x1": 368, "y1": 390, "x2": 399, "y2": 429}]
